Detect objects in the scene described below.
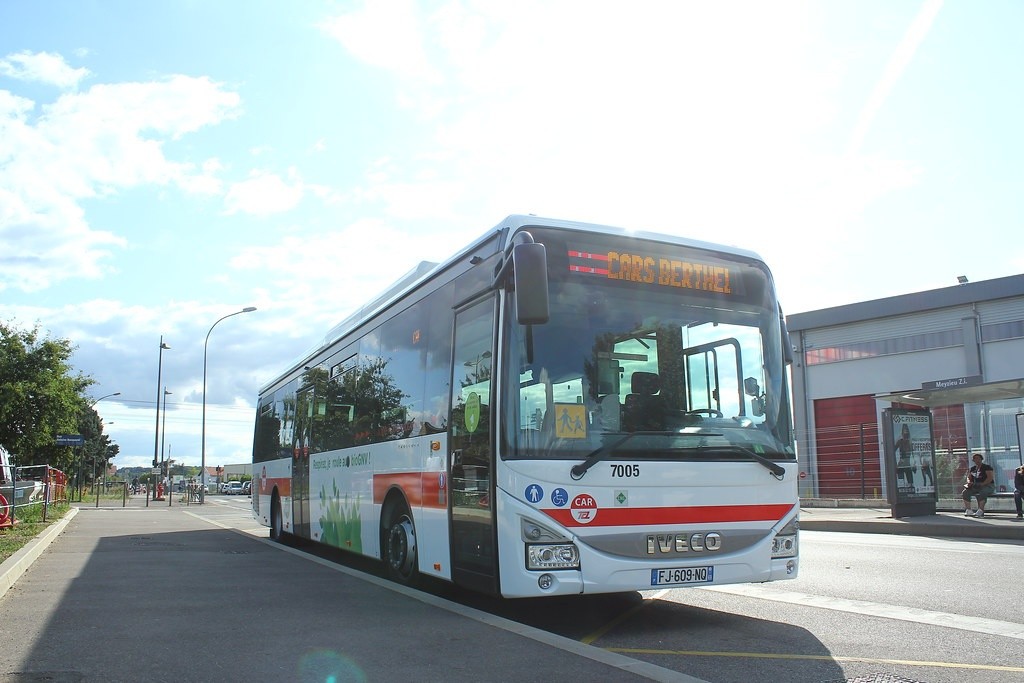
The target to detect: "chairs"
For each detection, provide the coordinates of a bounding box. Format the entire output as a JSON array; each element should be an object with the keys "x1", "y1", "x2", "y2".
[{"x1": 625, "y1": 372, "x2": 667, "y2": 433}]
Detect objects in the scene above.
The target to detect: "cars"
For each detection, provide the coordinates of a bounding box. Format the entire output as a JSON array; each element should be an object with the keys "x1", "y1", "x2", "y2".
[
  {"x1": 240, "y1": 481, "x2": 251, "y2": 494},
  {"x1": 244, "y1": 483, "x2": 251, "y2": 495},
  {"x1": 139, "y1": 487, "x2": 146, "y2": 493},
  {"x1": 222, "y1": 485, "x2": 229, "y2": 493},
  {"x1": 199, "y1": 485, "x2": 208, "y2": 493},
  {"x1": 136, "y1": 487, "x2": 141, "y2": 492}
]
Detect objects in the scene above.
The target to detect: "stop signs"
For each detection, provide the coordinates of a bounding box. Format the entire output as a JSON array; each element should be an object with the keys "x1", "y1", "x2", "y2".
[{"x1": 799, "y1": 471, "x2": 807, "y2": 479}]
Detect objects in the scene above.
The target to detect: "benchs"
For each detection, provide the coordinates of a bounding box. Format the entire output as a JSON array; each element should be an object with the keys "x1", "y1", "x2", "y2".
[{"x1": 980, "y1": 493, "x2": 1015, "y2": 516}]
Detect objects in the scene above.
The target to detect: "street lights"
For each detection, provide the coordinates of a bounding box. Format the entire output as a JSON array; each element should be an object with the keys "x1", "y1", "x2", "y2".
[{"x1": 200, "y1": 307, "x2": 257, "y2": 502}]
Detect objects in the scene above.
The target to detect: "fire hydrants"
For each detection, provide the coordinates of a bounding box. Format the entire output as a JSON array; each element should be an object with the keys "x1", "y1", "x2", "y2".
[{"x1": 158, "y1": 484, "x2": 164, "y2": 495}]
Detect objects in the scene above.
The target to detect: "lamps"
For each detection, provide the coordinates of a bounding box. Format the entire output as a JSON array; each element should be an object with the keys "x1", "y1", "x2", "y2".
[{"x1": 957, "y1": 276, "x2": 968, "y2": 286}]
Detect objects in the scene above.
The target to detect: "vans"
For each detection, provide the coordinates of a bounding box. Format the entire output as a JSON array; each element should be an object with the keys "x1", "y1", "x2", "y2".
[{"x1": 228, "y1": 482, "x2": 243, "y2": 493}]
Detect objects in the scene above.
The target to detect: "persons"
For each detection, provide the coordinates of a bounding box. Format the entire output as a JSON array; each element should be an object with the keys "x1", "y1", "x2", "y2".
[
  {"x1": 1011, "y1": 464, "x2": 1024, "y2": 523},
  {"x1": 157, "y1": 481, "x2": 169, "y2": 498},
  {"x1": 132, "y1": 476, "x2": 140, "y2": 495},
  {"x1": 190, "y1": 476, "x2": 195, "y2": 499},
  {"x1": 895, "y1": 425, "x2": 917, "y2": 490},
  {"x1": 919, "y1": 452, "x2": 934, "y2": 487},
  {"x1": 962, "y1": 454, "x2": 995, "y2": 518}
]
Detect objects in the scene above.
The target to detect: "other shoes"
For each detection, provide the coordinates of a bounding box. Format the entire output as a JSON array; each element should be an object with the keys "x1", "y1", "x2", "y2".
[
  {"x1": 964, "y1": 508, "x2": 974, "y2": 516},
  {"x1": 973, "y1": 509, "x2": 984, "y2": 518},
  {"x1": 1017, "y1": 511, "x2": 1023, "y2": 520}
]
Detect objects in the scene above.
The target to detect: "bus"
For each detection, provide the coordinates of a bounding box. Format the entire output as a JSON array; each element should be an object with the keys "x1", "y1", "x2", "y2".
[{"x1": 252, "y1": 213, "x2": 798, "y2": 599}]
